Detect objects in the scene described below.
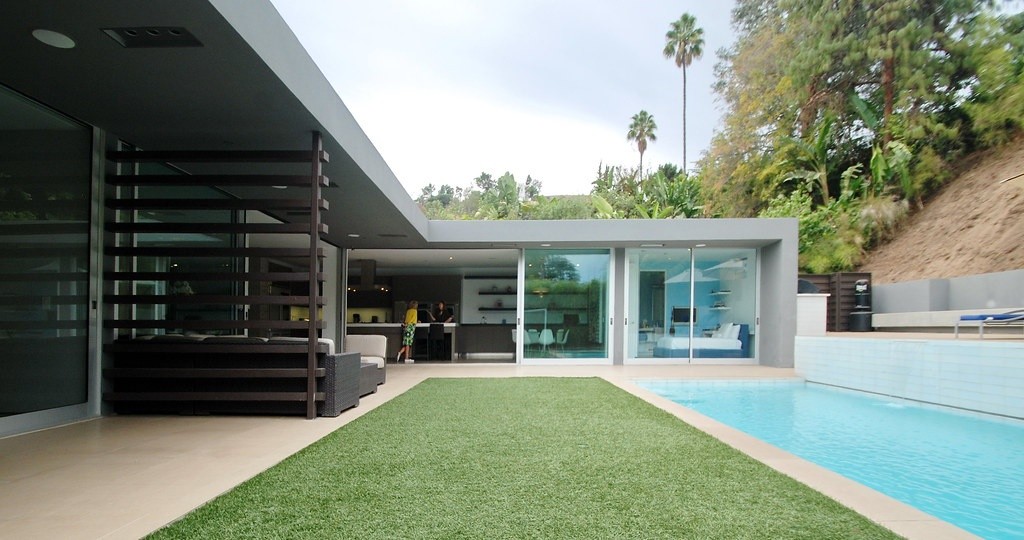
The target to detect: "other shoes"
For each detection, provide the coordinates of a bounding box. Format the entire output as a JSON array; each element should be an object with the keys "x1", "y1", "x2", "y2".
[
  {"x1": 397, "y1": 352, "x2": 401, "y2": 362},
  {"x1": 405, "y1": 359, "x2": 414, "y2": 364}
]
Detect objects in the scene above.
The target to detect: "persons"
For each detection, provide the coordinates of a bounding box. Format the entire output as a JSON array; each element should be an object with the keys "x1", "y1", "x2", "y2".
[
  {"x1": 397, "y1": 300, "x2": 418, "y2": 363},
  {"x1": 425, "y1": 300, "x2": 455, "y2": 323}
]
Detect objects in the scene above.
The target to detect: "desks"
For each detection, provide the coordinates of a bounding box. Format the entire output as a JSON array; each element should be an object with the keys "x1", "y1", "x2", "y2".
[{"x1": 702, "y1": 330, "x2": 715, "y2": 337}]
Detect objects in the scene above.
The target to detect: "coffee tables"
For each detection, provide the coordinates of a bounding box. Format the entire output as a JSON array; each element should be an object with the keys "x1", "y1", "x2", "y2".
[{"x1": 360, "y1": 362, "x2": 377, "y2": 397}]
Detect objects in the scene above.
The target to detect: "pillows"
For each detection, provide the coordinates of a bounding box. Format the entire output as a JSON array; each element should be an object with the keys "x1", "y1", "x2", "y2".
[
  {"x1": 729, "y1": 325, "x2": 741, "y2": 339},
  {"x1": 719, "y1": 323, "x2": 733, "y2": 338}
]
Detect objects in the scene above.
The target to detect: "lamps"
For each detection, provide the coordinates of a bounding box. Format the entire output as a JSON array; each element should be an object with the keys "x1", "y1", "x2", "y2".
[{"x1": 531, "y1": 278, "x2": 550, "y2": 298}]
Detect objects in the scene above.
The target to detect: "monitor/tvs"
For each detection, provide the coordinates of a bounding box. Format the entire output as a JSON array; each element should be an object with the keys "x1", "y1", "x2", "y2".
[{"x1": 672, "y1": 306, "x2": 698, "y2": 325}]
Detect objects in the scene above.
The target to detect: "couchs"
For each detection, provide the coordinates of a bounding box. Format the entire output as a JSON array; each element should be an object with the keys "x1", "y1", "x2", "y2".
[
  {"x1": 113, "y1": 334, "x2": 361, "y2": 417},
  {"x1": 345, "y1": 335, "x2": 388, "y2": 385}
]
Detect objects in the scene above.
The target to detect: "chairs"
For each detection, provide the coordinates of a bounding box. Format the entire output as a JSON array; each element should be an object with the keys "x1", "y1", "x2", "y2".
[
  {"x1": 512, "y1": 329, "x2": 569, "y2": 360},
  {"x1": 427, "y1": 324, "x2": 445, "y2": 360},
  {"x1": 955, "y1": 309, "x2": 1024, "y2": 338}
]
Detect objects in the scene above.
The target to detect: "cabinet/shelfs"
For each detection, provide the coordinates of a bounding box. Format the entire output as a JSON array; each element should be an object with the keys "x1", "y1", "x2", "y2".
[
  {"x1": 709, "y1": 292, "x2": 731, "y2": 310},
  {"x1": 478, "y1": 291, "x2": 588, "y2": 313}
]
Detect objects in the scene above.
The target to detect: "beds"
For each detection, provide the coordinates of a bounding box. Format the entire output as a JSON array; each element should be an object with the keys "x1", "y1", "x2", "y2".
[{"x1": 653, "y1": 324, "x2": 749, "y2": 358}]
